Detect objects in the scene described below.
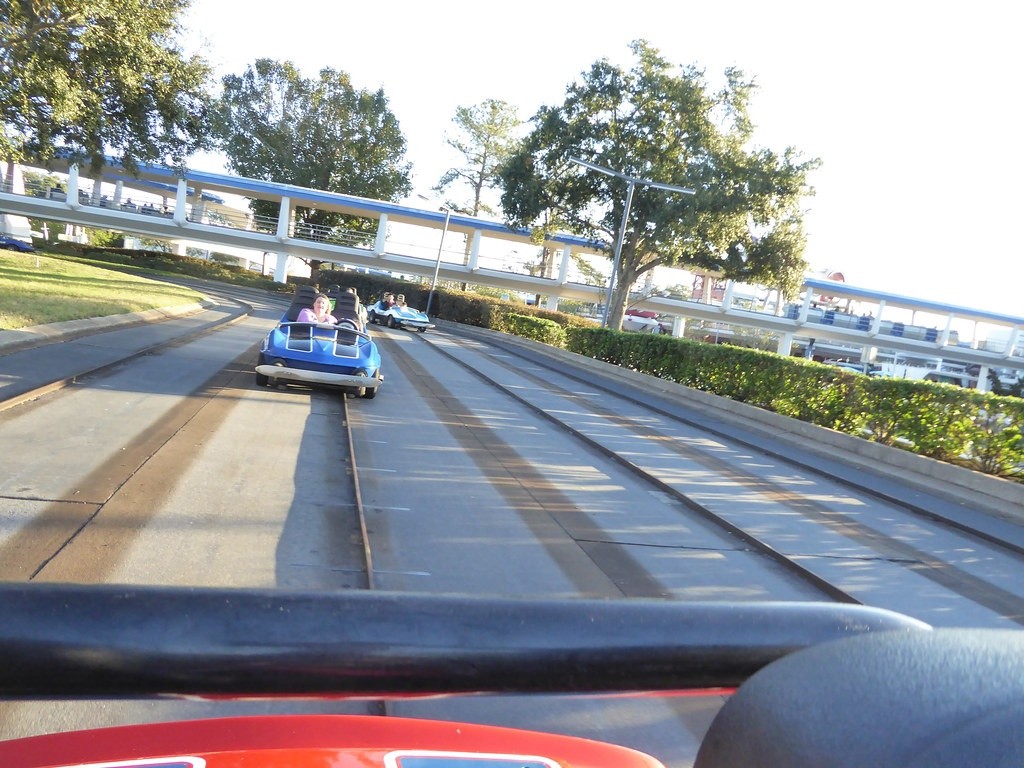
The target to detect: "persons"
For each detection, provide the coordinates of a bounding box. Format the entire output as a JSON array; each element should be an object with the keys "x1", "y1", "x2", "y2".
[
  {"x1": 51, "y1": 183, "x2": 169, "y2": 217},
  {"x1": 382, "y1": 291, "x2": 407, "y2": 311},
  {"x1": 296, "y1": 293, "x2": 338, "y2": 326}
]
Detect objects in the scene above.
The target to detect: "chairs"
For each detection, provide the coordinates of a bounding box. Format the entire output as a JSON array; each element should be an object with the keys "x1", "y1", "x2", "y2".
[
  {"x1": 328, "y1": 283, "x2": 340, "y2": 293},
  {"x1": 381, "y1": 291, "x2": 391, "y2": 304},
  {"x1": 351, "y1": 286, "x2": 358, "y2": 296},
  {"x1": 50, "y1": 188, "x2": 65, "y2": 200},
  {"x1": 332, "y1": 291, "x2": 362, "y2": 324},
  {"x1": 288, "y1": 285, "x2": 320, "y2": 321},
  {"x1": 121, "y1": 204, "x2": 139, "y2": 213},
  {"x1": 397, "y1": 293, "x2": 405, "y2": 305}
]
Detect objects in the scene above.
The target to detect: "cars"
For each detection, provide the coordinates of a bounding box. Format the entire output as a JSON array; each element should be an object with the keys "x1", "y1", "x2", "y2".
[
  {"x1": 0, "y1": 234, "x2": 36, "y2": 254},
  {"x1": 625, "y1": 310, "x2": 660, "y2": 320},
  {"x1": 366, "y1": 300, "x2": 435, "y2": 333},
  {"x1": 254, "y1": 284, "x2": 384, "y2": 399}
]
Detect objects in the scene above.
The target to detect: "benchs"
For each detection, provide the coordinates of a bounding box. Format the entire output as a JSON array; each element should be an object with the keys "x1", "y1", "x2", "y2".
[{"x1": 786, "y1": 305, "x2": 959, "y2": 347}]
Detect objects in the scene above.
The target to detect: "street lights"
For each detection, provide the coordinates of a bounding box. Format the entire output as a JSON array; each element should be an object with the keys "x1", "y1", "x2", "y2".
[{"x1": 568, "y1": 156, "x2": 697, "y2": 329}]
